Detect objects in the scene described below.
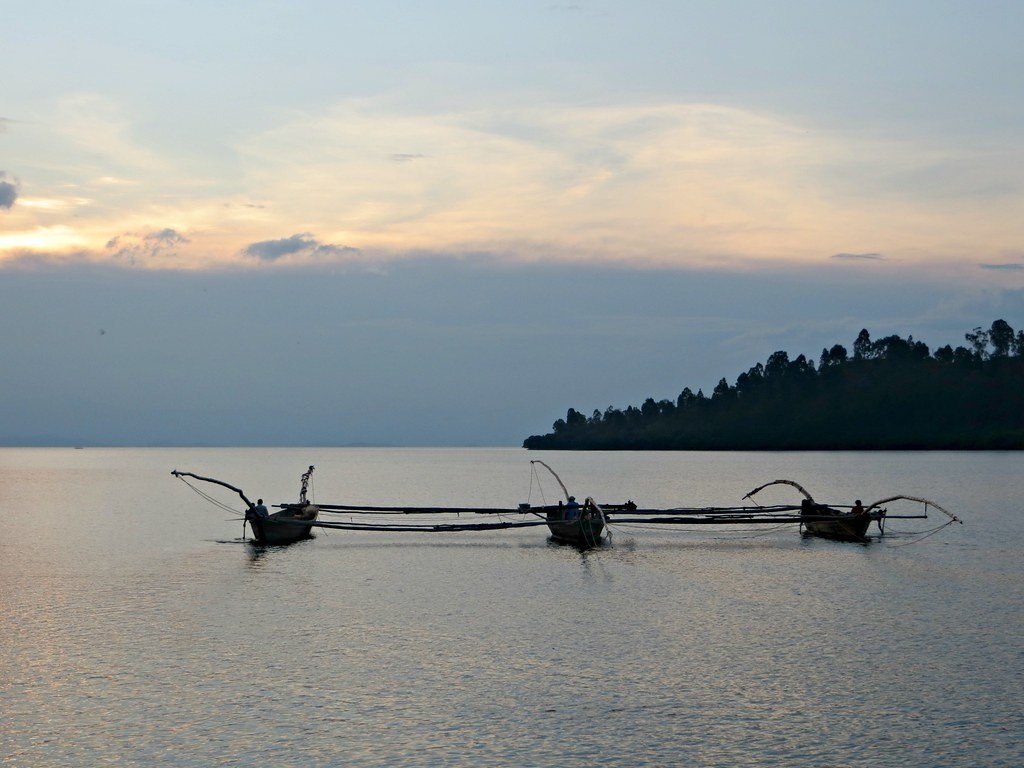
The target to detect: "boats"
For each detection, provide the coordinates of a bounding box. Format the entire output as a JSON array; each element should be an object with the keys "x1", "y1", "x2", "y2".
[
  {"x1": 743, "y1": 479, "x2": 963, "y2": 542},
  {"x1": 530, "y1": 459, "x2": 611, "y2": 545},
  {"x1": 172, "y1": 462, "x2": 320, "y2": 546}
]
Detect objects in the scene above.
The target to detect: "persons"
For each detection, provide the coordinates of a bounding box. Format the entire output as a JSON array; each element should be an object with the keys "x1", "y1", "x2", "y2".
[
  {"x1": 565, "y1": 496, "x2": 579, "y2": 520},
  {"x1": 851, "y1": 500, "x2": 864, "y2": 513},
  {"x1": 255, "y1": 499, "x2": 271, "y2": 520}
]
